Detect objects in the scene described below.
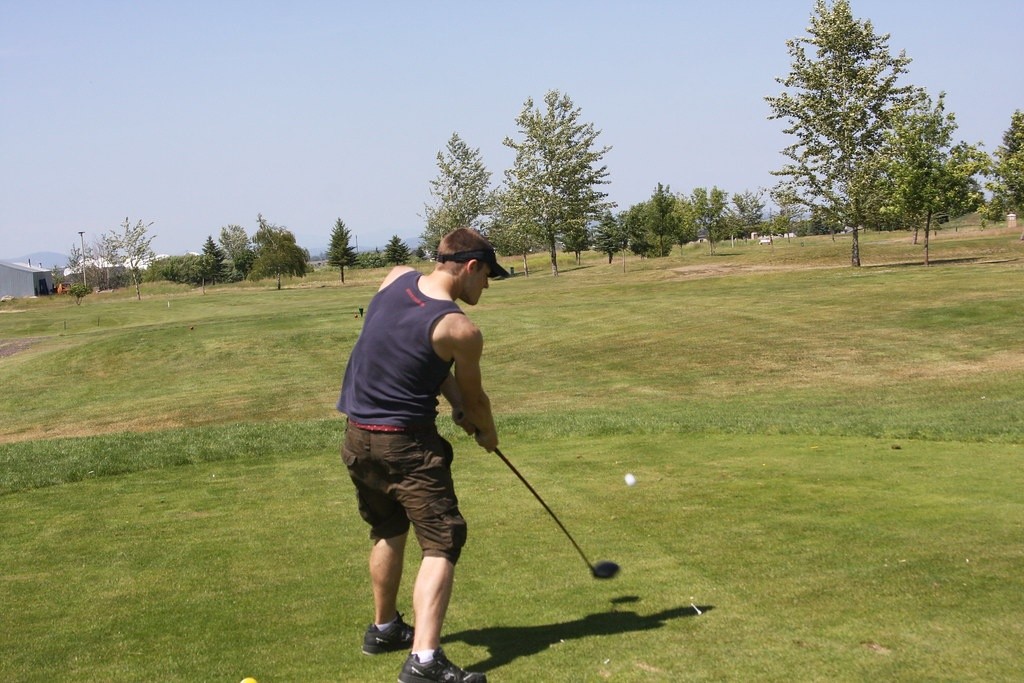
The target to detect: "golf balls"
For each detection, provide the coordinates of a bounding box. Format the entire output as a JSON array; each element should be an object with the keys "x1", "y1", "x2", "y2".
[{"x1": 625, "y1": 473, "x2": 637, "y2": 486}]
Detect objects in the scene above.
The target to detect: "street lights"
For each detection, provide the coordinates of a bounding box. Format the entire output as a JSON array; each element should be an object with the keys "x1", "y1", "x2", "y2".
[{"x1": 78, "y1": 232, "x2": 86, "y2": 288}]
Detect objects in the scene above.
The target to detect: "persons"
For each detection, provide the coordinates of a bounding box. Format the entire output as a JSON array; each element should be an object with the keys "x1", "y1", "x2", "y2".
[{"x1": 335, "y1": 226, "x2": 510, "y2": 683}]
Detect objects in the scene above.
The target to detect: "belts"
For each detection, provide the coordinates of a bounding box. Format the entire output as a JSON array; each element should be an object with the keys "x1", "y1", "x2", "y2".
[{"x1": 348, "y1": 417, "x2": 410, "y2": 432}]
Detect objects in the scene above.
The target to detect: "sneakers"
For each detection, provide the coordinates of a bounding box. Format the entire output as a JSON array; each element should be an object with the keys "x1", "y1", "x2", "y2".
[
  {"x1": 362, "y1": 609, "x2": 415, "y2": 655},
  {"x1": 397, "y1": 647, "x2": 487, "y2": 683}
]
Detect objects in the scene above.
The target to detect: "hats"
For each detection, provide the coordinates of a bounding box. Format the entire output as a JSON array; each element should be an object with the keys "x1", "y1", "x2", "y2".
[{"x1": 435, "y1": 248, "x2": 510, "y2": 278}]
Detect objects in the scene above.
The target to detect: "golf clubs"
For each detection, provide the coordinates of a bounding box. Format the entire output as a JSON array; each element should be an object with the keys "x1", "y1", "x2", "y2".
[{"x1": 458, "y1": 410, "x2": 621, "y2": 578}]
